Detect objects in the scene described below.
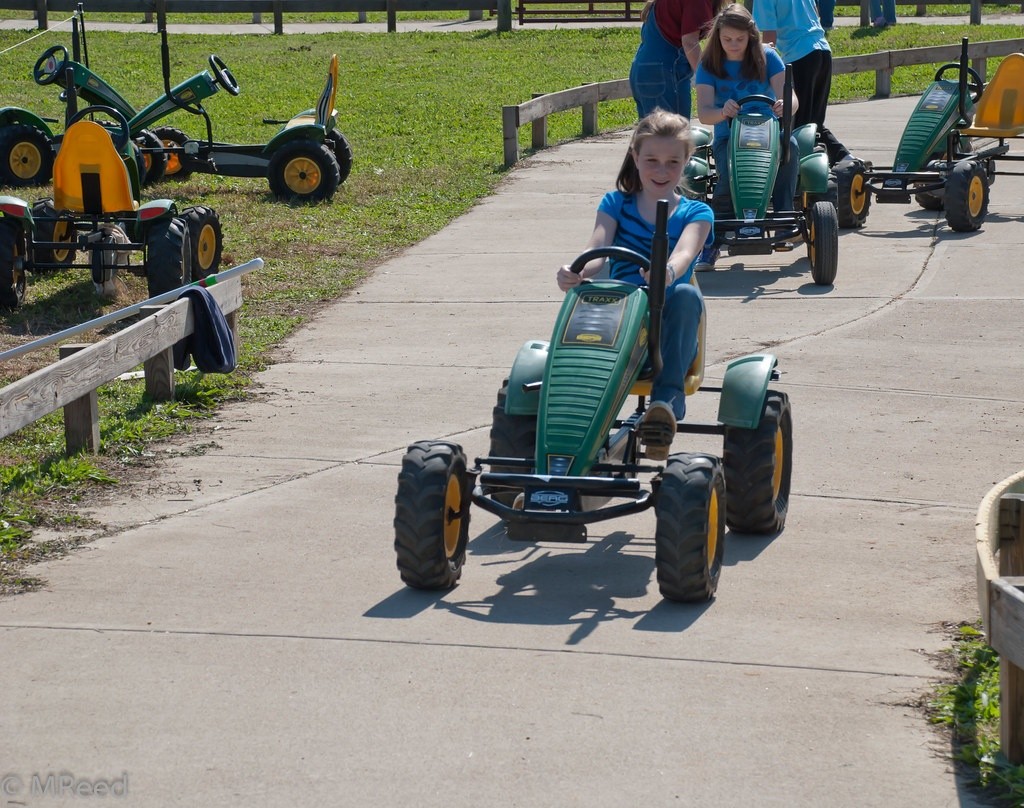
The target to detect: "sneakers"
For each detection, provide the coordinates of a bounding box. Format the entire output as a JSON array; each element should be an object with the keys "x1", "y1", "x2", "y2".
[
  {"x1": 775, "y1": 240, "x2": 793, "y2": 252},
  {"x1": 695, "y1": 245, "x2": 720, "y2": 271}
]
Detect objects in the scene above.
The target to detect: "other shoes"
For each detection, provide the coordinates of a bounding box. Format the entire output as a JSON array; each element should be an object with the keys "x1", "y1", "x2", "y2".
[
  {"x1": 822, "y1": 26, "x2": 834, "y2": 32},
  {"x1": 887, "y1": 22, "x2": 896, "y2": 27},
  {"x1": 512, "y1": 492, "x2": 526, "y2": 510},
  {"x1": 874, "y1": 17, "x2": 886, "y2": 27},
  {"x1": 643, "y1": 400, "x2": 677, "y2": 461}
]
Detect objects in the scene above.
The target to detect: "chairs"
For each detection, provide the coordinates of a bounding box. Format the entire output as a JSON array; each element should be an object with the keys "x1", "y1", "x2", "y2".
[
  {"x1": 285, "y1": 54, "x2": 339, "y2": 131},
  {"x1": 954, "y1": 53, "x2": 1024, "y2": 138},
  {"x1": 51, "y1": 120, "x2": 138, "y2": 214},
  {"x1": 628, "y1": 271, "x2": 707, "y2": 396}
]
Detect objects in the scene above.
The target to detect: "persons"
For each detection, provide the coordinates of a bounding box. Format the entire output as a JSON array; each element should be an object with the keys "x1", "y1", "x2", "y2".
[
  {"x1": 694, "y1": 3, "x2": 800, "y2": 270},
  {"x1": 629, "y1": 0, "x2": 729, "y2": 121},
  {"x1": 815, "y1": 0, "x2": 836, "y2": 29},
  {"x1": 751, "y1": 0, "x2": 873, "y2": 167},
  {"x1": 870, "y1": 0, "x2": 896, "y2": 27},
  {"x1": 512, "y1": 107, "x2": 715, "y2": 512}
]
